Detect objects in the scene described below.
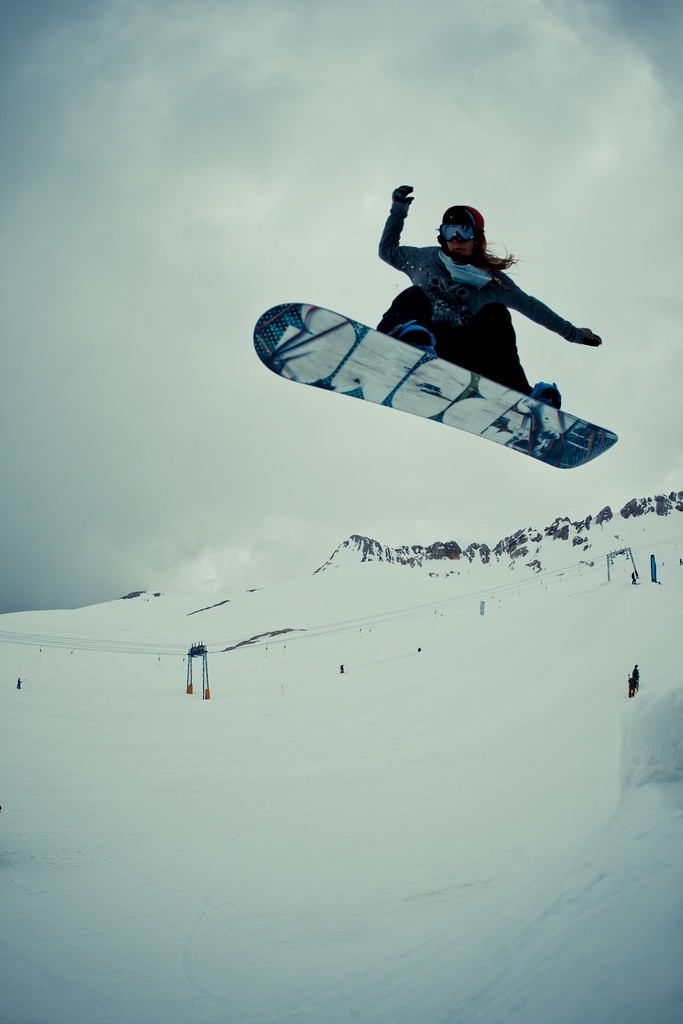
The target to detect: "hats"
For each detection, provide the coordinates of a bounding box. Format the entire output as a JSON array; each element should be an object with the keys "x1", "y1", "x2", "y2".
[{"x1": 441, "y1": 205, "x2": 484, "y2": 237}]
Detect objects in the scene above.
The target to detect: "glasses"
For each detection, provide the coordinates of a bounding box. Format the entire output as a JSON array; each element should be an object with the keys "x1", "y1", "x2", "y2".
[{"x1": 441, "y1": 223, "x2": 474, "y2": 242}]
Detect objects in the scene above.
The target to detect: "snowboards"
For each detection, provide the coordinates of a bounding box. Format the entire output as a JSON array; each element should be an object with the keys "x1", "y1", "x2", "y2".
[{"x1": 253, "y1": 303, "x2": 618, "y2": 469}]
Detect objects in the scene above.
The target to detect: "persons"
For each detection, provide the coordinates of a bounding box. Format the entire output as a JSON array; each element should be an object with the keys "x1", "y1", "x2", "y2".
[
  {"x1": 680, "y1": 559, "x2": 682, "y2": 565},
  {"x1": 378, "y1": 186, "x2": 602, "y2": 410},
  {"x1": 631, "y1": 572, "x2": 637, "y2": 584},
  {"x1": 628, "y1": 665, "x2": 640, "y2": 698}
]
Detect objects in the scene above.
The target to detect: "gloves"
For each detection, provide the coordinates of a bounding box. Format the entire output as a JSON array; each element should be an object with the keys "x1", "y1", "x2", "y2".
[
  {"x1": 581, "y1": 328, "x2": 602, "y2": 346},
  {"x1": 392, "y1": 185, "x2": 414, "y2": 204}
]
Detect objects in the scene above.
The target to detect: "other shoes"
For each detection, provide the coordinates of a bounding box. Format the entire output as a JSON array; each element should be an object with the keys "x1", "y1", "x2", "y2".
[
  {"x1": 529, "y1": 383, "x2": 562, "y2": 407},
  {"x1": 397, "y1": 323, "x2": 433, "y2": 352}
]
